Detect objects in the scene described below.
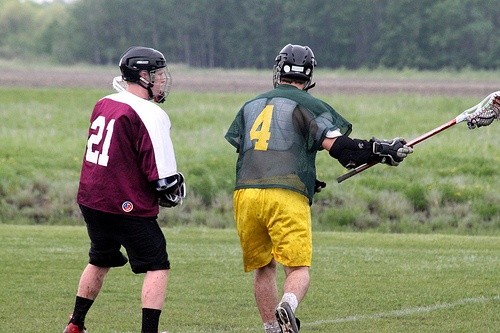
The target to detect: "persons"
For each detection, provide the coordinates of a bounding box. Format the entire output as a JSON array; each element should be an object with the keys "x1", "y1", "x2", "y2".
[
  {"x1": 225, "y1": 43, "x2": 413, "y2": 333},
  {"x1": 61, "y1": 47, "x2": 186, "y2": 333}
]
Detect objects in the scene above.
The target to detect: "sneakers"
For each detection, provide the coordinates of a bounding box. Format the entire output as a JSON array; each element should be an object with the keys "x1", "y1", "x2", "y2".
[{"x1": 275, "y1": 301, "x2": 298, "y2": 333}]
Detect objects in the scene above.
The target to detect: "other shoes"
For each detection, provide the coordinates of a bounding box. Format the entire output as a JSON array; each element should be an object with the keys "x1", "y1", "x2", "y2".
[{"x1": 62, "y1": 322, "x2": 85, "y2": 333}]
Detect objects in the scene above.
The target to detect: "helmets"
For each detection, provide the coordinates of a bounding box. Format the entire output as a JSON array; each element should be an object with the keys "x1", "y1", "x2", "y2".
[
  {"x1": 118, "y1": 45, "x2": 166, "y2": 83},
  {"x1": 273, "y1": 43, "x2": 315, "y2": 80}
]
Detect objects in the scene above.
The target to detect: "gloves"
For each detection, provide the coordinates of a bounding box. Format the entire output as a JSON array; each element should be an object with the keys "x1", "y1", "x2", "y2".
[
  {"x1": 156, "y1": 175, "x2": 187, "y2": 209},
  {"x1": 370, "y1": 136, "x2": 411, "y2": 167},
  {"x1": 313, "y1": 179, "x2": 325, "y2": 194}
]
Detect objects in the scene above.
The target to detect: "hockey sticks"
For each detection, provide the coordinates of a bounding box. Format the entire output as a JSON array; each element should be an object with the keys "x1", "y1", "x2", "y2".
[
  {"x1": 336, "y1": 89, "x2": 500, "y2": 183},
  {"x1": 112, "y1": 75, "x2": 127, "y2": 92}
]
